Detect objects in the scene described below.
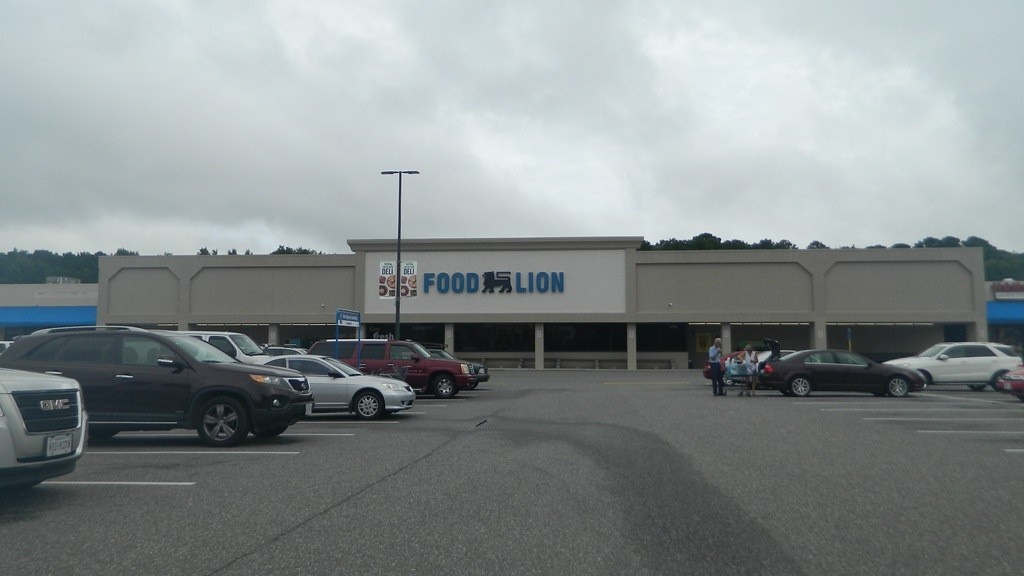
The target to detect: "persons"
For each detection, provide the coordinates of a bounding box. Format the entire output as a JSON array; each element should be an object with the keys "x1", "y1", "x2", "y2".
[
  {"x1": 738, "y1": 344, "x2": 759, "y2": 396},
  {"x1": 708, "y1": 338, "x2": 725, "y2": 396}
]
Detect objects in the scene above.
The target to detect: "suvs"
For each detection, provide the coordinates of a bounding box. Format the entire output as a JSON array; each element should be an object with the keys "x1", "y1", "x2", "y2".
[
  {"x1": 0, "y1": 324, "x2": 316, "y2": 448},
  {"x1": 304, "y1": 338, "x2": 479, "y2": 400}
]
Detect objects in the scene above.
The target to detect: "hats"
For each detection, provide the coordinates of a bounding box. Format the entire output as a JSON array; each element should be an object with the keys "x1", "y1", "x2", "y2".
[{"x1": 746, "y1": 344, "x2": 753, "y2": 351}]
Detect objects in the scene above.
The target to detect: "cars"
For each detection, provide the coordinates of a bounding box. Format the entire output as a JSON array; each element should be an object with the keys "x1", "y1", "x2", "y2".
[
  {"x1": 400, "y1": 349, "x2": 490, "y2": 391},
  {"x1": 245, "y1": 354, "x2": 417, "y2": 422},
  {"x1": 0, "y1": 366, "x2": 90, "y2": 489},
  {"x1": 881, "y1": 341, "x2": 1024, "y2": 393},
  {"x1": 703, "y1": 350, "x2": 822, "y2": 392},
  {"x1": 0, "y1": 330, "x2": 311, "y2": 364},
  {"x1": 758, "y1": 339, "x2": 926, "y2": 399},
  {"x1": 994, "y1": 363, "x2": 1024, "y2": 400}
]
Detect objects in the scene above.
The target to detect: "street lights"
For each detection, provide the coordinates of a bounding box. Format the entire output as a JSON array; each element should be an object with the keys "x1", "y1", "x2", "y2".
[{"x1": 381, "y1": 171, "x2": 420, "y2": 342}]
